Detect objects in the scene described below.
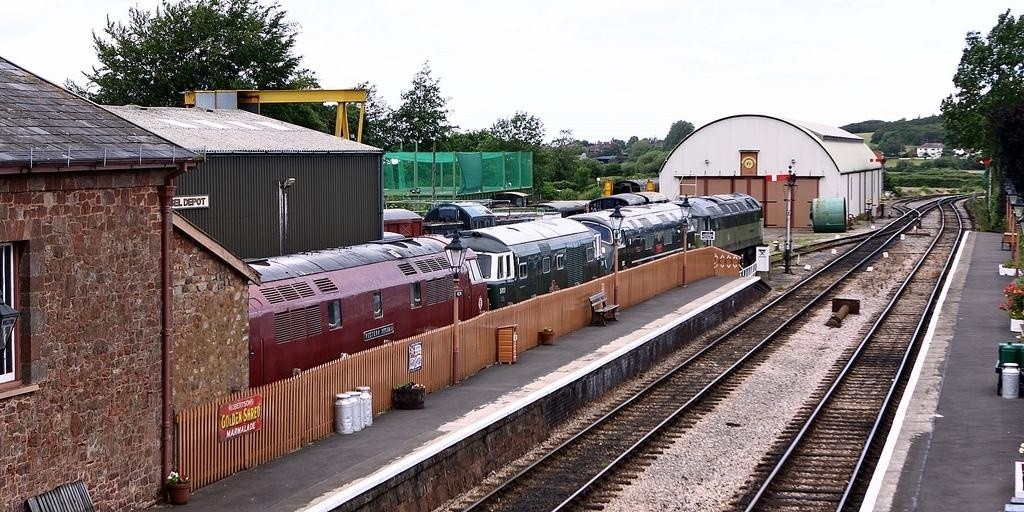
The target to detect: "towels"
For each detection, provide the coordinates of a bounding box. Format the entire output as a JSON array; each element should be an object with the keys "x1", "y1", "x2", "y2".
[
  {"x1": 999, "y1": 343, "x2": 1024, "y2": 398},
  {"x1": 497, "y1": 325, "x2": 517, "y2": 366}
]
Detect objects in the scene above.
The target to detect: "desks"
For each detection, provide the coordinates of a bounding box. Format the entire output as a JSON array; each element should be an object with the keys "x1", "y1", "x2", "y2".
[
  {"x1": 444, "y1": 234, "x2": 467, "y2": 387},
  {"x1": 782, "y1": 166, "x2": 795, "y2": 271},
  {"x1": 609, "y1": 205, "x2": 625, "y2": 315},
  {"x1": 680, "y1": 194, "x2": 691, "y2": 288}
]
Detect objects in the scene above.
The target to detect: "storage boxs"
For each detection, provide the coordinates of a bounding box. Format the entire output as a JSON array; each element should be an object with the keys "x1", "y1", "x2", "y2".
[{"x1": 584, "y1": 290, "x2": 620, "y2": 328}]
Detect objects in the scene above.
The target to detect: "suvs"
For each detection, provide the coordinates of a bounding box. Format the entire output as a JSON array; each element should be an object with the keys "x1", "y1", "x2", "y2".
[
  {"x1": 1001, "y1": 342, "x2": 1021, "y2": 401},
  {"x1": 334, "y1": 387, "x2": 372, "y2": 434}
]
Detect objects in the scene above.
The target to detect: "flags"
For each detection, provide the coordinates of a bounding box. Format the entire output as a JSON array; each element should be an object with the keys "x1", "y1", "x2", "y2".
[
  {"x1": 169, "y1": 483, "x2": 190, "y2": 505},
  {"x1": 536, "y1": 330, "x2": 554, "y2": 344}
]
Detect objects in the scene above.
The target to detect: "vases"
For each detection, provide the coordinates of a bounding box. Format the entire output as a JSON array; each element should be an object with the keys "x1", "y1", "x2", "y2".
[
  {"x1": 167, "y1": 471, "x2": 188, "y2": 484},
  {"x1": 542, "y1": 325, "x2": 552, "y2": 332}
]
